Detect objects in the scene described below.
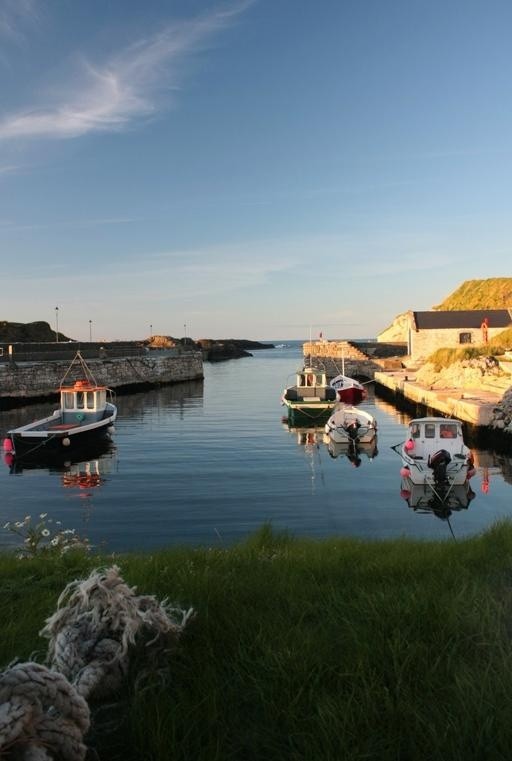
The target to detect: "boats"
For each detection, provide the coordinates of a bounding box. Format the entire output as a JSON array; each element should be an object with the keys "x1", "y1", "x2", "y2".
[
  {"x1": 8, "y1": 434, "x2": 116, "y2": 489},
  {"x1": 401, "y1": 417, "x2": 473, "y2": 485},
  {"x1": 282, "y1": 366, "x2": 377, "y2": 444},
  {"x1": 7, "y1": 350, "x2": 117, "y2": 455},
  {"x1": 400, "y1": 476, "x2": 476, "y2": 521},
  {"x1": 281, "y1": 416, "x2": 377, "y2": 496}
]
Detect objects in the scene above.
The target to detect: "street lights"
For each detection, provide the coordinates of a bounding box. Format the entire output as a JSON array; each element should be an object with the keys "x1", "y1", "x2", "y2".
[
  {"x1": 184, "y1": 324, "x2": 187, "y2": 345},
  {"x1": 54, "y1": 306, "x2": 59, "y2": 342},
  {"x1": 89, "y1": 319, "x2": 92, "y2": 342},
  {"x1": 150, "y1": 325, "x2": 153, "y2": 337}
]
